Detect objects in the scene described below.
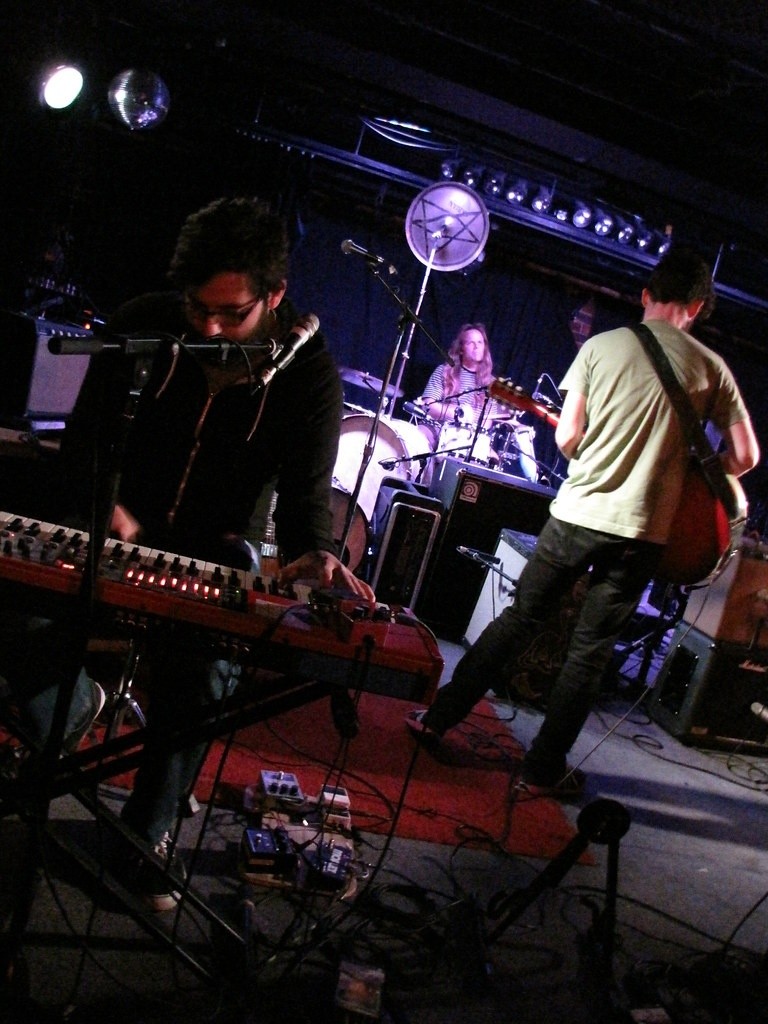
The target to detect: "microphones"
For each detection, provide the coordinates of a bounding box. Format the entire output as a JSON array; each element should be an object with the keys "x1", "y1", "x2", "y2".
[
  {"x1": 532, "y1": 377, "x2": 545, "y2": 401},
  {"x1": 253, "y1": 313, "x2": 320, "y2": 393},
  {"x1": 458, "y1": 544, "x2": 501, "y2": 565},
  {"x1": 402, "y1": 401, "x2": 440, "y2": 429},
  {"x1": 341, "y1": 239, "x2": 395, "y2": 270},
  {"x1": 750, "y1": 702, "x2": 768, "y2": 722},
  {"x1": 379, "y1": 462, "x2": 395, "y2": 471}
]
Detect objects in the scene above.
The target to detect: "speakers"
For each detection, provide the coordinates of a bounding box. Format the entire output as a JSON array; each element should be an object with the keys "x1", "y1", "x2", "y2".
[
  {"x1": 0, "y1": 309, "x2": 96, "y2": 421},
  {"x1": 646, "y1": 620, "x2": 768, "y2": 758},
  {"x1": 683, "y1": 536, "x2": 768, "y2": 652},
  {"x1": 415, "y1": 456, "x2": 559, "y2": 651}
]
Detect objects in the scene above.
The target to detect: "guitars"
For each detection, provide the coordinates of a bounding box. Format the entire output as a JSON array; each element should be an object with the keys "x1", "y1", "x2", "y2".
[{"x1": 485, "y1": 373, "x2": 749, "y2": 592}]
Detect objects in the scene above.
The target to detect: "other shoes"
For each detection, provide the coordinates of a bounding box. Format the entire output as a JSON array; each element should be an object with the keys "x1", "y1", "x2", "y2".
[
  {"x1": 535, "y1": 762, "x2": 589, "y2": 790},
  {"x1": 401, "y1": 707, "x2": 445, "y2": 736},
  {"x1": 21, "y1": 681, "x2": 108, "y2": 776}
]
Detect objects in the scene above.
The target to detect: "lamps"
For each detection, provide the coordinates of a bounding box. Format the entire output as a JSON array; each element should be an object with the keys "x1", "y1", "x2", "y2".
[{"x1": 440, "y1": 153, "x2": 672, "y2": 254}]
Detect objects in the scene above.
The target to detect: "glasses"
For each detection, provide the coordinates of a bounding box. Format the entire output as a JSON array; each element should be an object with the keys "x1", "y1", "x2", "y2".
[{"x1": 187, "y1": 282, "x2": 277, "y2": 325}]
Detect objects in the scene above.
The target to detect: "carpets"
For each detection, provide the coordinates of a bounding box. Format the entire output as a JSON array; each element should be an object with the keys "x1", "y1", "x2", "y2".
[{"x1": 1, "y1": 640, "x2": 599, "y2": 864}]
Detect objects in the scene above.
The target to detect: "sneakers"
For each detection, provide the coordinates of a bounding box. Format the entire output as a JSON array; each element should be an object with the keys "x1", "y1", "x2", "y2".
[{"x1": 137, "y1": 829, "x2": 188, "y2": 912}]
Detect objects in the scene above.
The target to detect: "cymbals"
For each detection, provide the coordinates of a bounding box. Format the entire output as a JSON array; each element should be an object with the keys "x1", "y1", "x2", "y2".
[
  {"x1": 338, "y1": 364, "x2": 405, "y2": 398},
  {"x1": 493, "y1": 418, "x2": 521, "y2": 427}
]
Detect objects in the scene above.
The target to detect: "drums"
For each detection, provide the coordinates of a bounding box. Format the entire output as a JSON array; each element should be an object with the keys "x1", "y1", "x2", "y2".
[
  {"x1": 435, "y1": 420, "x2": 492, "y2": 469},
  {"x1": 277, "y1": 477, "x2": 371, "y2": 575},
  {"x1": 332, "y1": 413, "x2": 437, "y2": 522}
]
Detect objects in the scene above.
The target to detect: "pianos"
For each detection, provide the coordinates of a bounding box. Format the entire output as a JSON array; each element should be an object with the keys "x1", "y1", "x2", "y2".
[{"x1": 3, "y1": 500, "x2": 447, "y2": 1023}]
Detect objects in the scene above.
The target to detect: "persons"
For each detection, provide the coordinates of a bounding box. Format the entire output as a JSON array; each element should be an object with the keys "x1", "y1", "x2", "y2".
[
  {"x1": 0, "y1": 197, "x2": 376, "y2": 910},
  {"x1": 416, "y1": 325, "x2": 517, "y2": 486},
  {"x1": 405, "y1": 250, "x2": 760, "y2": 800}
]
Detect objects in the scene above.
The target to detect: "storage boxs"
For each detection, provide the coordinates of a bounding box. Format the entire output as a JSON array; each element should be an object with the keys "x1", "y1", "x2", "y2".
[{"x1": 684, "y1": 552, "x2": 768, "y2": 651}]
